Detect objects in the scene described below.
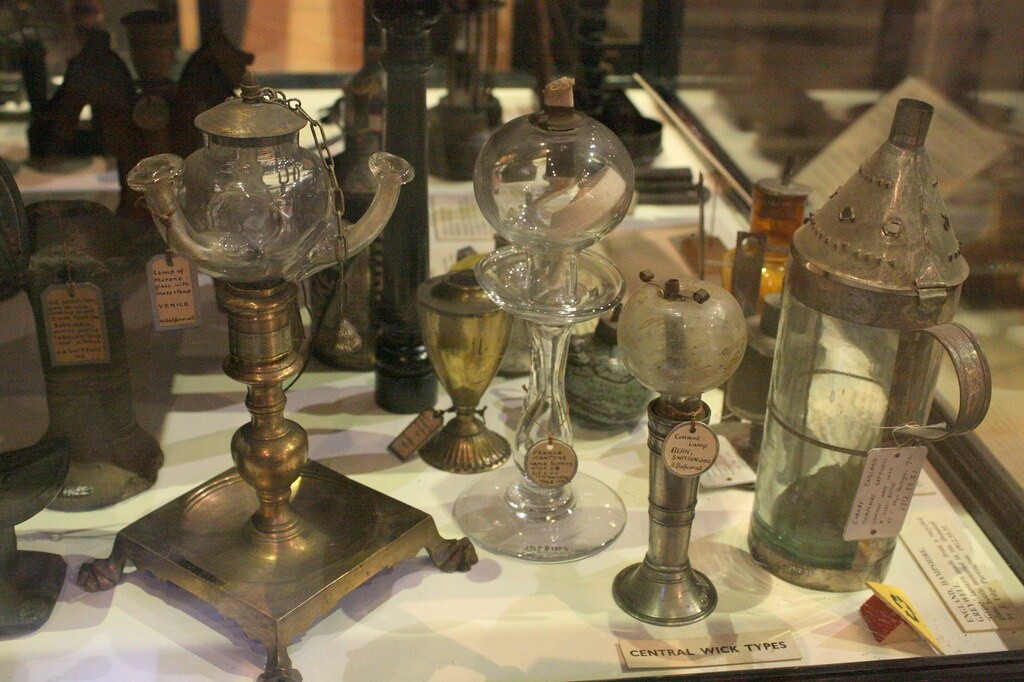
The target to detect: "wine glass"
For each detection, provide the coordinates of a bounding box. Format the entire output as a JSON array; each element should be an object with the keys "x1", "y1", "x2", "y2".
[{"x1": 416, "y1": 272, "x2": 512, "y2": 474}]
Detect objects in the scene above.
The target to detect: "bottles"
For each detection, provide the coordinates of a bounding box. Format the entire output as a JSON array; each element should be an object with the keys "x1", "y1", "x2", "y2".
[
  {"x1": 727, "y1": 178, "x2": 810, "y2": 426},
  {"x1": 760, "y1": 99, "x2": 992, "y2": 591}
]
